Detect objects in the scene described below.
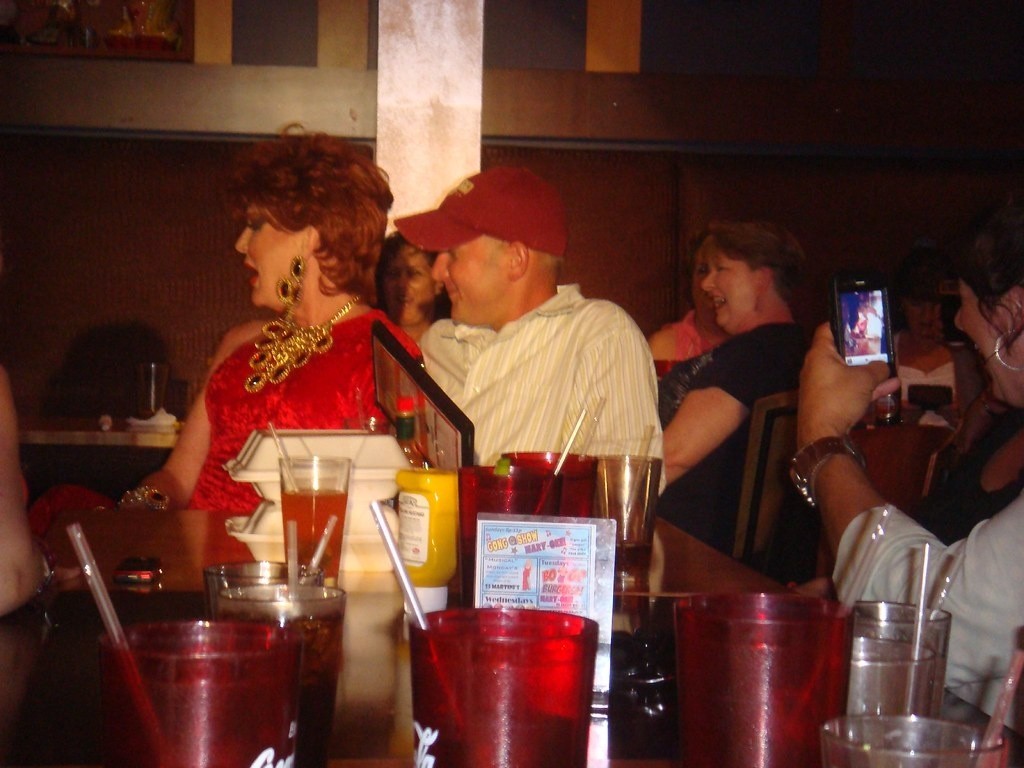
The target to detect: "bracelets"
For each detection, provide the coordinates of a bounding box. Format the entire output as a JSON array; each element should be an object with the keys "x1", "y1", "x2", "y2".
[
  {"x1": 38, "y1": 543, "x2": 64, "y2": 590},
  {"x1": 980, "y1": 392, "x2": 998, "y2": 417}
]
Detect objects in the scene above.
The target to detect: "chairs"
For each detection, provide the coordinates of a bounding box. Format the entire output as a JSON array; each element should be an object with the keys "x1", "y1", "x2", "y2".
[
  {"x1": 818, "y1": 417, "x2": 967, "y2": 574},
  {"x1": 734, "y1": 389, "x2": 799, "y2": 562}
]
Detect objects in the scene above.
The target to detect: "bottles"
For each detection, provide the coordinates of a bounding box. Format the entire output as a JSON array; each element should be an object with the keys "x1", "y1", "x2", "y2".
[
  {"x1": 395, "y1": 468, "x2": 460, "y2": 614},
  {"x1": 877, "y1": 362, "x2": 901, "y2": 425},
  {"x1": 393, "y1": 399, "x2": 424, "y2": 510}
]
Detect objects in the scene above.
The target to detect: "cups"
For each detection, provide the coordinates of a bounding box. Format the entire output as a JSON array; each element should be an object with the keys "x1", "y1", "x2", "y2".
[
  {"x1": 99, "y1": 620, "x2": 297, "y2": 768},
  {"x1": 460, "y1": 453, "x2": 661, "y2": 592},
  {"x1": 140, "y1": 362, "x2": 168, "y2": 419},
  {"x1": 408, "y1": 608, "x2": 599, "y2": 768},
  {"x1": 818, "y1": 602, "x2": 1011, "y2": 767},
  {"x1": 278, "y1": 456, "x2": 351, "y2": 589},
  {"x1": 670, "y1": 598, "x2": 848, "y2": 768},
  {"x1": 201, "y1": 561, "x2": 346, "y2": 768}
]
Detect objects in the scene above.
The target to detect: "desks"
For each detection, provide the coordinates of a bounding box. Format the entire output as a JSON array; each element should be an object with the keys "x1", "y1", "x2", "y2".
[
  {"x1": 0, "y1": 509, "x2": 1024, "y2": 768},
  {"x1": 19, "y1": 414, "x2": 182, "y2": 448}
]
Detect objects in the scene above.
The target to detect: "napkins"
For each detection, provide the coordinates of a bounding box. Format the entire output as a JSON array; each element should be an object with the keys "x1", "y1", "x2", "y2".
[{"x1": 127, "y1": 408, "x2": 176, "y2": 425}]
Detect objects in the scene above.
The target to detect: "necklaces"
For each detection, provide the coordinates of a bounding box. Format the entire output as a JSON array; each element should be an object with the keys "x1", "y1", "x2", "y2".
[{"x1": 245, "y1": 296, "x2": 361, "y2": 392}]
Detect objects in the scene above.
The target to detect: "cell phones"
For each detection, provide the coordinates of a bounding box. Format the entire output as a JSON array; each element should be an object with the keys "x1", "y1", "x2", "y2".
[
  {"x1": 111, "y1": 555, "x2": 166, "y2": 584},
  {"x1": 828, "y1": 267, "x2": 901, "y2": 428}
]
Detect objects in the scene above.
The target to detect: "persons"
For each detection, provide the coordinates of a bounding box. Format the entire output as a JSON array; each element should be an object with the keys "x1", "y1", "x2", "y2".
[
  {"x1": 0, "y1": 361, "x2": 78, "y2": 617},
  {"x1": 376, "y1": 231, "x2": 452, "y2": 351},
  {"x1": 396, "y1": 165, "x2": 669, "y2": 503},
  {"x1": 118, "y1": 131, "x2": 474, "y2": 515},
  {"x1": 637, "y1": 197, "x2": 1024, "y2": 738}
]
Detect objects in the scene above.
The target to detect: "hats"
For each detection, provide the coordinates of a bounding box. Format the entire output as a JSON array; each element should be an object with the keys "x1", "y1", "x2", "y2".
[{"x1": 393, "y1": 168, "x2": 568, "y2": 256}]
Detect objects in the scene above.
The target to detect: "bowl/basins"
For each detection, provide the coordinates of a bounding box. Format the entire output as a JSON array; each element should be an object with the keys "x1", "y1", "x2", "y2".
[
  {"x1": 225, "y1": 503, "x2": 400, "y2": 571},
  {"x1": 225, "y1": 428, "x2": 413, "y2": 503}
]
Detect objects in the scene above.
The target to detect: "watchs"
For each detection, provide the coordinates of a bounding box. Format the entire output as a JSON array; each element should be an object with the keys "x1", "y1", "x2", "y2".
[
  {"x1": 138, "y1": 480, "x2": 169, "y2": 511},
  {"x1": 788, "y1": 435, "x2": 866, "y2": 506}
]
